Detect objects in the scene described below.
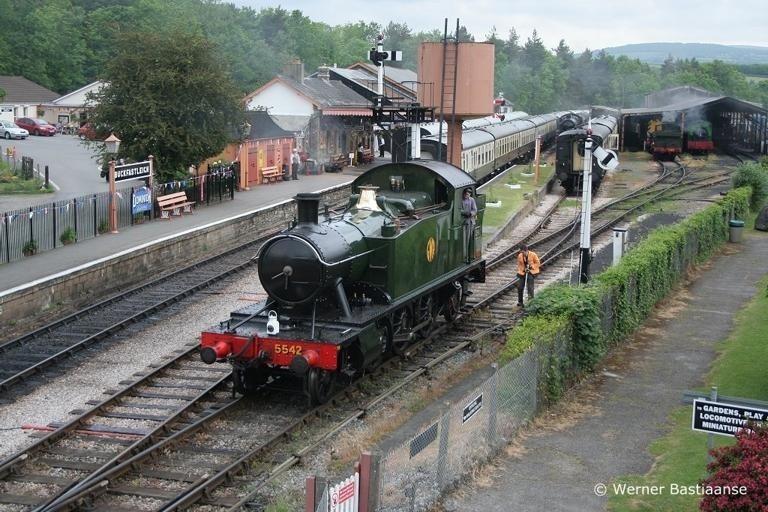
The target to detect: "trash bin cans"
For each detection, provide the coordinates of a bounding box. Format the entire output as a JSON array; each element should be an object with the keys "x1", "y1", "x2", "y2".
[
  {"x1": 282, "y1": 164, "x2": 289, "y2": 181},
  {"x1": 729, "y1": 220, "x2": 744, "y2": 243}
]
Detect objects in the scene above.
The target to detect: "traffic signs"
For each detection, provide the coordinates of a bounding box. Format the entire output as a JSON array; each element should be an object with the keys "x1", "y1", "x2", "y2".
[{"x1": 693, "y1": 400, "x2": 768, "y2": 437}]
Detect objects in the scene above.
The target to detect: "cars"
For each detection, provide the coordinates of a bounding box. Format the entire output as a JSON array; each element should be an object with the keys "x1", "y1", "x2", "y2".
[
  {"x1": 16, "y1": 118, "x2": 55, "y2": 136},
  {"x1": 0, "y1": 120, "x2": 29, "y2": 139},
  {"x1": 78, "y1": 122, "x2": 113, "y2": 141}
]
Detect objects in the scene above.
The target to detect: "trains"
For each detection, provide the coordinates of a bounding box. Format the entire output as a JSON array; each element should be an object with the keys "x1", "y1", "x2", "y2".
[
  {"x1": 628, "y1": 116, "x2": 683, "y2": 161},
  {"x1": 676, "y1": 117, "x2": 716, "y2": 154},
  {"x1": 391, "y1": 108, "x2": 621, "y2": 191},
  {"x1": 199, "y1": 159, "x2": 484, "y2": 404}
]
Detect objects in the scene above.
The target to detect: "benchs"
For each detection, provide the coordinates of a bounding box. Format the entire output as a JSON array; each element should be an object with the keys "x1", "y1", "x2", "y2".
[
  {"x1": 261, "y1": 166, "x2": 286, "y2": 183},
  {"x1": 330, "y1": 154, "x2": 351, "y2": 165},
  {"x1": 156, "y1": 191, "x2": 196, "y2": 220},
  {"x1": 364, "y1": 149, "x2": 374, "y2": 161}
]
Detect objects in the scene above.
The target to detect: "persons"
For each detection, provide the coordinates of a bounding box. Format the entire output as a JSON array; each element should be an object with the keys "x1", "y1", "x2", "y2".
[
  {"x1": 289, "y1": 148, "x2": 301, "y2": 180},
  {"x1": 461, "y1": 188, "x2": 478, "y2": 264},
  {"x1": 356, "y1": 142, "x2": 365, "y2": 166},
  {"x1": 378, "y1": 135, "x2": 386, "y2": 158},
  {"x1": 513, "y1": 244, "x2": 541, "y2": 307}
]
